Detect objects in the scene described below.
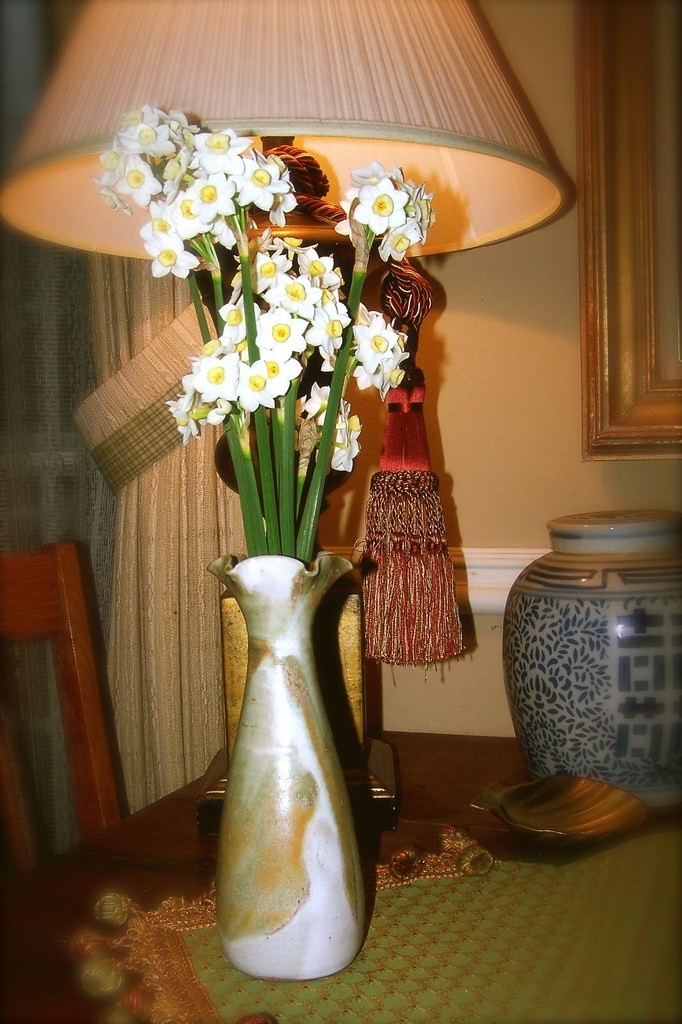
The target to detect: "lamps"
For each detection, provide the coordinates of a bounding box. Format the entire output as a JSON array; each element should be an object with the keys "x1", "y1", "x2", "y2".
[{"x1": 1, "y1": 0, "x2": 573, "y2": 833}]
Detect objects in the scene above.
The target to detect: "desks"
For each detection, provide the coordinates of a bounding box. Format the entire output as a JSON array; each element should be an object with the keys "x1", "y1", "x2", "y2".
[{"x1": 0, "y1": 729, "x2": 682, "y2": 1024}]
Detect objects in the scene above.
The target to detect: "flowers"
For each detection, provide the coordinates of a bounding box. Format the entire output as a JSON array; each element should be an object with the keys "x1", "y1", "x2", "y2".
[{"x1": 93, "y1": 105, "x2": 436, "y2": 567}]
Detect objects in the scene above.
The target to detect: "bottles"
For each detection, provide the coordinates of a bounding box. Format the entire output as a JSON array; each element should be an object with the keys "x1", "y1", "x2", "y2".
[{"x1": 501, "y1": 504, "x2": 682, "y2": 807}]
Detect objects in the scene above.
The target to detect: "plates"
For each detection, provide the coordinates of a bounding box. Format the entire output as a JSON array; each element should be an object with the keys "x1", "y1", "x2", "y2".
[{"x1": 467, "y1": 774, "x2": 650, "y2": 851}]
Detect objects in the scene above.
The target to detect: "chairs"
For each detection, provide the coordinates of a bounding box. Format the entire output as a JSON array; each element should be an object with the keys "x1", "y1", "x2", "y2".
[{"x1": 0, "y1": 540, "x2": 125, "y2": 878}]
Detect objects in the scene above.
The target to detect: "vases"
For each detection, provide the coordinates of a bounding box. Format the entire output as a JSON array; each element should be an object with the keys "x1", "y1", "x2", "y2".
[{"x1": 204, "y1": 550, "x2": 367, "y2": 980}]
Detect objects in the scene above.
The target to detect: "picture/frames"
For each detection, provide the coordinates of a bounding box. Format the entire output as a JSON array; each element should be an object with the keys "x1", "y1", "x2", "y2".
[{"x1": 571, "y1": 0, "x2": 682, "y2": 464}]
